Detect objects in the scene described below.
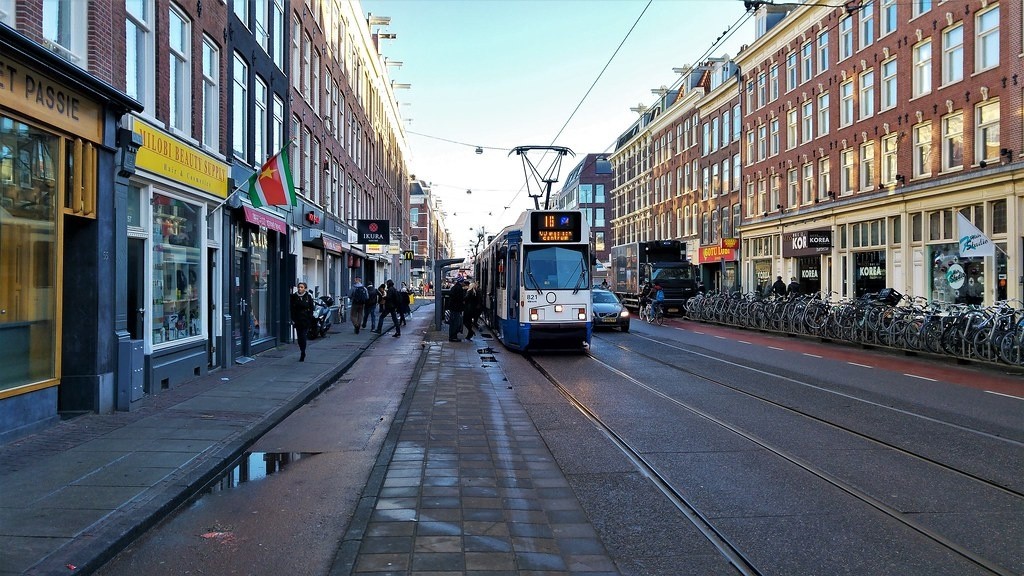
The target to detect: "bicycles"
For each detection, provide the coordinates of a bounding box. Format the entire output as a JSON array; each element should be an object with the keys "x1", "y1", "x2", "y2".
[
  {"x1": 637, "y1": 294, "x2": 665, "y2": 326},
  {"x1": 685, "y1": 288, "x2": 1024, "y2": 372}
]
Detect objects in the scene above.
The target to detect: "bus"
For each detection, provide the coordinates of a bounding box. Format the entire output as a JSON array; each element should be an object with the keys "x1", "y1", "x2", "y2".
[{"x1": 469, "y1": 209, "x2": 593, "y2": 354}]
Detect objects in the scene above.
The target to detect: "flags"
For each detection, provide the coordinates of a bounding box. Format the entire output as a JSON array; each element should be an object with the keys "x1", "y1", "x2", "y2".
[
  {"x1": 248, "y1": 141, "x2": 297, "y2": 208},
  {"x1": 957, "y1": 212, "x2": 996, "y2": 257}
]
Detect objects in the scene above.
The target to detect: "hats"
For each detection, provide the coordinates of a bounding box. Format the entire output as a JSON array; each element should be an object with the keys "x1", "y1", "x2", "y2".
[{"x1": 457, "y1": 276, "x2": 464, "y2": 282}]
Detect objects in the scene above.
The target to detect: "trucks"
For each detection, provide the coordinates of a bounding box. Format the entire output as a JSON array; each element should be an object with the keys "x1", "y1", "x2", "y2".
[{"x1": 609, "y1": 238, "x2": 697, "y2": 318}]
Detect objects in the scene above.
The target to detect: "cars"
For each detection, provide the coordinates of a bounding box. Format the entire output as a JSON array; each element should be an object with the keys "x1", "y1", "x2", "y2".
[{"x1": 589, "y1": 288, "x2": 632, "y2": 332}]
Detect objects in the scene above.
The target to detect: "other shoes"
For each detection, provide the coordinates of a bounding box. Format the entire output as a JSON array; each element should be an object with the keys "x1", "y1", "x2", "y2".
[
  {"x1": 354, "y1": 322, "x2": 406, "y2": 337},
  {"x1": 449, "y1": 321, "x2": 480, "y2": 342},
  {"x1": 299, "y1": 351, "x2": 306, "y2": 362},
  {"x1": 651, "y1": 311, "x2": 663, "y2": 322}
]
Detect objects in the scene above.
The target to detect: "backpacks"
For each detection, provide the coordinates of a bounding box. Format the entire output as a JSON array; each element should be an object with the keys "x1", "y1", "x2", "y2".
[
  {"x1": 656, "y1": 289, "x2": 664, "y2": 302},
  {"x1": 353, "y1": 285, "x2": 367, "y2": 305}
]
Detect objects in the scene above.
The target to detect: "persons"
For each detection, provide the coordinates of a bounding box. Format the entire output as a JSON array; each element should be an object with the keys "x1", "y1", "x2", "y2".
[
  {"x1": 757, "y1": 275, "x2": 801, "y2": 300},
  {"x1": 462, "y1": 280, "x2": 476, "y2": 340},
  {"x1": 290, "y1": 282, "x2": 315, "y2": 362},
  {"x1": 602, "y1": 279, "x2": 608, "y2": 286},
  {"x1": 473, "y1": 279, "x2": 484, "y2": 332},
  {"x1": 348, "y1": 276, "x2": 370, "y2": 334},
  {"x1": 371, "y1": 280, "x2": 401, "y2": 337},
  {"x1": 449, "y1": 277, "x2": 467, "y2": 342},
  {"x1": 362, "y1": 281, "x2": 377, "y2": 332},
  {"x1": 399, "y1": 282, "x2": 414, "y2": 320},
  {"x1": 649, "y1": 285, "x2": 664, "y2": 322},
  {"x1": 410, "y1": 280, "x2": 433, "y2": 299},
  {"x1": 640, "y1": 281, "x2": 653, "y2": 309},
  {"x1": 397, "y1": 287, "x2": 410, "y2": 326}
]
